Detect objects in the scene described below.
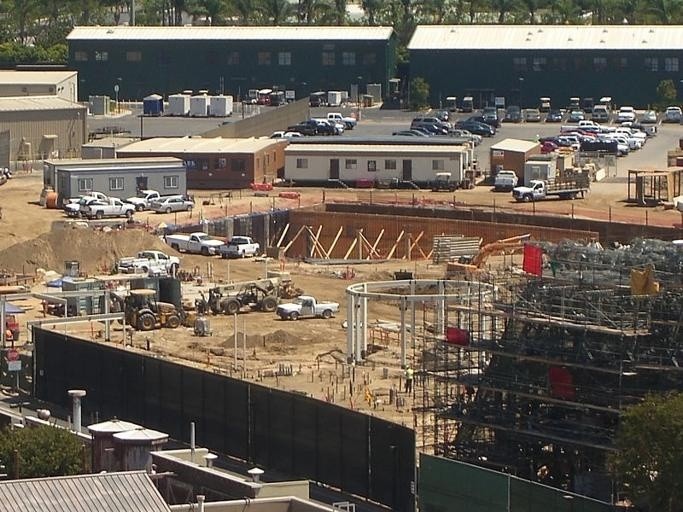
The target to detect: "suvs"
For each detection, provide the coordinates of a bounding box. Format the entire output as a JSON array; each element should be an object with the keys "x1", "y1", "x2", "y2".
[
  {"x1": 208, "y1": 282, "x2": 276, "y2": 317},
  {"x1": 391, "y1": 94, "x2": 682, "y2": 159},
  {"x1": 259, "y1": 112, "x2": 358, "y2": 139},
  {"x1": 4, "y1": 315, "x2": 19, "y2": 340}
]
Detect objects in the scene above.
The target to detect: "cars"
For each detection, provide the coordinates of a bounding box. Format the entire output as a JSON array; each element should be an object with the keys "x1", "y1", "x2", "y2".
[
  {"x1": 0, "y1": 168, "x2": 7, "y2": 185},
  {"x1": 151, "y1": 196, "x2": 194, "y2": 214}
]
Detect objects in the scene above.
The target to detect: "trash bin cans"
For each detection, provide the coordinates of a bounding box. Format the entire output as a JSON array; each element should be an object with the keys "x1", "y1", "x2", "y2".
[{"x1": 461, "y1": 178, "x2": 470, "y2": 189}]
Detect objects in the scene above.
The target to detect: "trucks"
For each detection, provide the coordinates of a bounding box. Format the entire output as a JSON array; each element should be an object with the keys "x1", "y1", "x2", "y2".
[
  {"x1": 512, "y1": 178, "x2": 587, "y2": 201},
  {"x1": 247, "y1": 87, "x2": 349, "y2": 108}
]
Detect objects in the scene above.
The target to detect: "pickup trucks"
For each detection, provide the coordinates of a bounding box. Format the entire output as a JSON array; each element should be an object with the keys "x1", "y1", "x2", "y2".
[
  {"x1": 66, "y1": 197, "x2": 101, "y2": 216},
  {"x1": 218, "y1": 235, "x2": 259, "y2": 259},
  {"x1": 89, "y1": 124, "x2": 130, "y2": 141},
  {"x1": 61, "y1": 191, "x2": 110, "y2": 209},
  {"x1": 85, "y1": 197, "x2": 136, "y2": 218},
  {"x1": 275, "y1": 296, "x2": 340, "y2": 321},
  {"x1": 116, "y1": 250, "x2": 180, "y2": 276},
  {"x1": 167, "y1": 232, "x2": 224, "y2": 256},
  {"x1": 125, "y1": 190, "x2": 179, "y2": 211},
  {"x1": 494, "y1": 169, "x2": 519, "y2": 192}
]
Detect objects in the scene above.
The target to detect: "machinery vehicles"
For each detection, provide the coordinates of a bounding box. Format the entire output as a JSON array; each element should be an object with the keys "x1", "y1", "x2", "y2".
[
  {"x1": 445, "y1": 231, "x2": 551, "y2": 280},
  {"x1": 118, "y1": 288, "x2": 199, "y2": 333}
]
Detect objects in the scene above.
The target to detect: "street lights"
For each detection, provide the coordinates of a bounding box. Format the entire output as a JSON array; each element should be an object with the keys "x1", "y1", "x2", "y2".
[
  {"x1": 111, "y1": 76, "x2": 122, "y2": 113},
  {"x1": 353, "y1": 75, "x2": 363, "y2": 121}
]
